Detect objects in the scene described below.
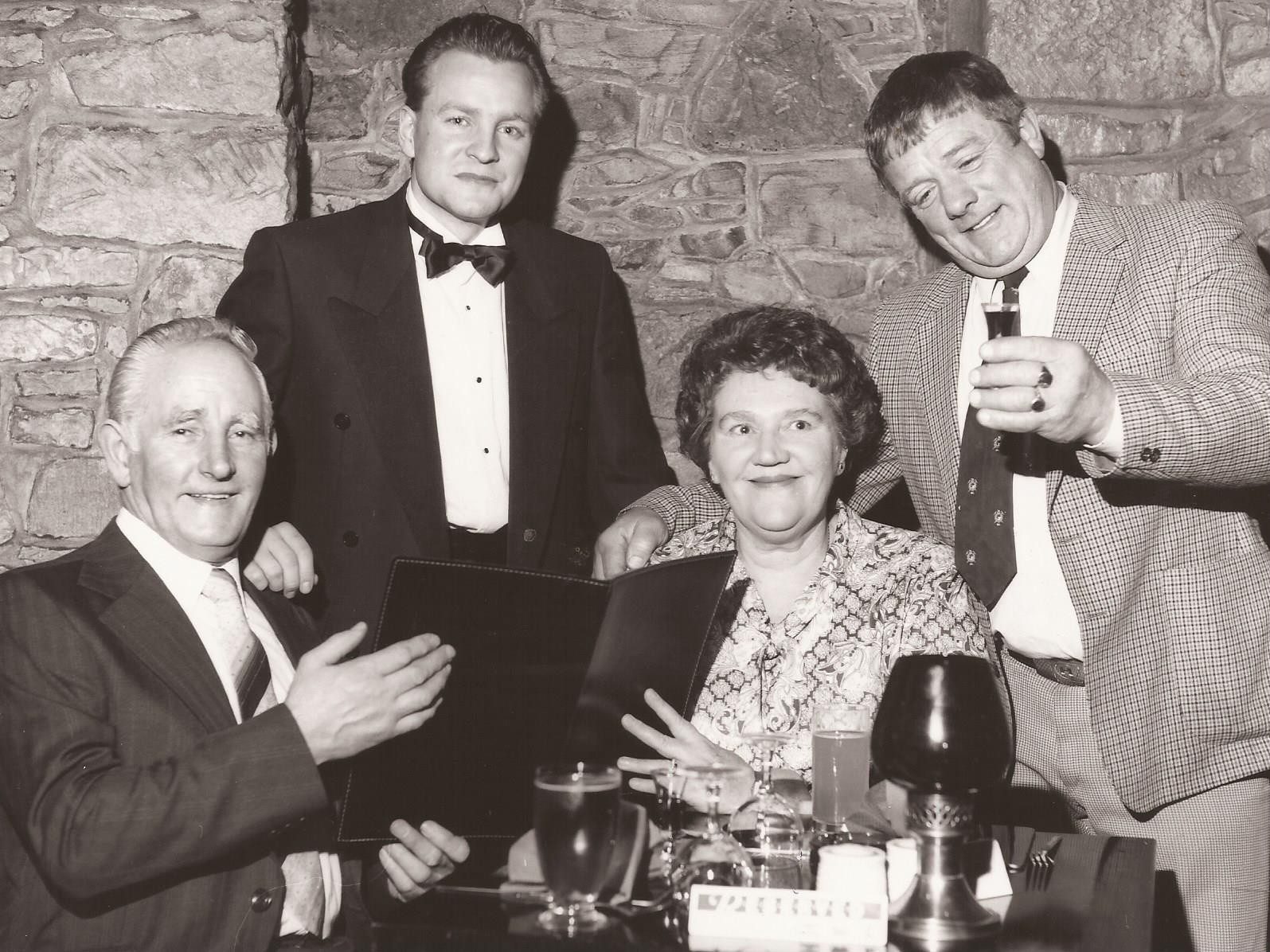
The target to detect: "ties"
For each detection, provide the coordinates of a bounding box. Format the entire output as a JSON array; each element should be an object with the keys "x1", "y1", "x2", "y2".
[
  {"x1": 201, "y1": 568, "x2": 325, "y2": 940},
  {"x1": 955, "y1": 266, "x2": 1030, "y2": 612}
]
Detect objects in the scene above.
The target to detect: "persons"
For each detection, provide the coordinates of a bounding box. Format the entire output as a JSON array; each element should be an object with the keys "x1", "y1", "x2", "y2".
[
  {"x1": 589, "y1": 307, "x2": 1016, "y2": 829},
  {"x1": 0, "y1": 315, "x2": 470, "y2": 952},
  {"x1": 591, "y1": 53, "x2": 1269, "y2": 952},
  {"x1": 216, "y1": 12, "x2": 678, "y2": 663}
]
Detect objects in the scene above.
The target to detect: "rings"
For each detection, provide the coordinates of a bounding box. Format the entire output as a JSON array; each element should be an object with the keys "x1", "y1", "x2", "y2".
[
  {"x1": 1033, "y1": 387, "x2": 1046, "y2": 413},
  {"x1": 1037, "y1": 362, "x2": 1052, "y2": 388}
]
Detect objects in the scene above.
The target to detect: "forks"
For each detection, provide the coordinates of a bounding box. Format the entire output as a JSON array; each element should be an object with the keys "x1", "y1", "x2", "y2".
[{"x1": 1027, "y1": 835, "x2": 1062, "y2": 868}]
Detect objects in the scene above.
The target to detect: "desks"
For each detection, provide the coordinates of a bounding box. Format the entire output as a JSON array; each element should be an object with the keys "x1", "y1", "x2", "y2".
[{"x1": 370, "y1": 824, "x2": 1157, "y2": 952}]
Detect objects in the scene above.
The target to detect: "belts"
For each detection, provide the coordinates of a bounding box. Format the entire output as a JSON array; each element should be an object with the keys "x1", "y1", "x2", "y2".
[{"x1": 993, "y1": 632, "x2": 1085, "y2": 687}]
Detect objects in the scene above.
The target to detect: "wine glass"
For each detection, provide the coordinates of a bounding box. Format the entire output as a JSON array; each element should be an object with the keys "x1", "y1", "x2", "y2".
[
  {"x1": 870, "y1": 653, "x2": 1017, "y2": 949},
  {"x1": 728, "y1": 731, "x2": 805, "y2": 871},
  {"x1": 535, "y1": 761, "x2": 622, "y2": 935},
  {"x1": 649, "y1": 766, "x2": 754, "y2": 945}
]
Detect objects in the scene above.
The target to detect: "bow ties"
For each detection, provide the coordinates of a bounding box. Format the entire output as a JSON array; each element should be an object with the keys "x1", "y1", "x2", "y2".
[{"x1": 417, "y1": 233, "x2": 516, "y2": 288}]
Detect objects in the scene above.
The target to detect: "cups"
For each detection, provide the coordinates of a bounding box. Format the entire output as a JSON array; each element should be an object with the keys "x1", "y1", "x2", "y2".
[{"x1": 810, "y1": 704, "x2": 873, "y2": 847}]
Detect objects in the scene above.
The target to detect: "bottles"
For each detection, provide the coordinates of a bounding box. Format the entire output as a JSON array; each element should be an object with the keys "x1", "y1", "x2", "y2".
[{"x1": 977, "y1": 301, "x2": 1021, "y2": 391}]
